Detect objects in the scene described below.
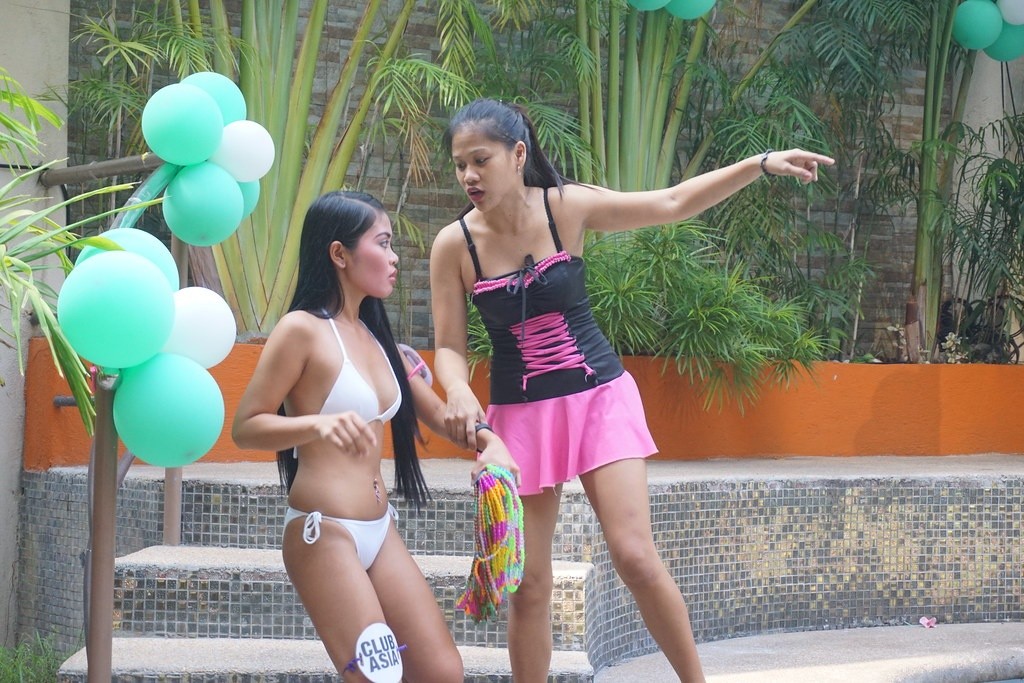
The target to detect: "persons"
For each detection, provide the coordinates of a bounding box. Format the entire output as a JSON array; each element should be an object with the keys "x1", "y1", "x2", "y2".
[
  {"x1": 431, "y1": 102, "x2": 834, "y2": 683},
  {"x1": 230, "y1": 192, "x2": 522, "y2": 683}
]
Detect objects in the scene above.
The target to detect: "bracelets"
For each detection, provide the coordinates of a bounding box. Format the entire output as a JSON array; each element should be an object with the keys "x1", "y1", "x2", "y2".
[
  {"x1": 761, "y1": 148, "x2": 777, "y2": 177},
  {"x1": 476, "y1": 423, "x2": 494, "y2": 454}
]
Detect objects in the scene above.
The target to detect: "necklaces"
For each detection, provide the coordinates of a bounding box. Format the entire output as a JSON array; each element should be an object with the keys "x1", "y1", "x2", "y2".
[{"x1": 456, "y1": 465, "x2": 525, "y2": 625}]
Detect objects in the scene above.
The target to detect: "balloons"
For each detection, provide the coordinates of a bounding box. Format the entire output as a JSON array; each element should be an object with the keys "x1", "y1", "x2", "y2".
[
  {"x1": 664, "y1": 0, "x2": 717, "y2": 20},
  {"x1": 951, "y1": 0, "x2": 1024, "y2": 62},
  {"x1": 57, "y1": 227, "x2": 237, "y2": 468},
  {"x1": 142, "y1": 71, "x2": 275, "y2": 247},
  {"x1": 627, "y1": 0, "x2": 671, "y2": 11}
]
